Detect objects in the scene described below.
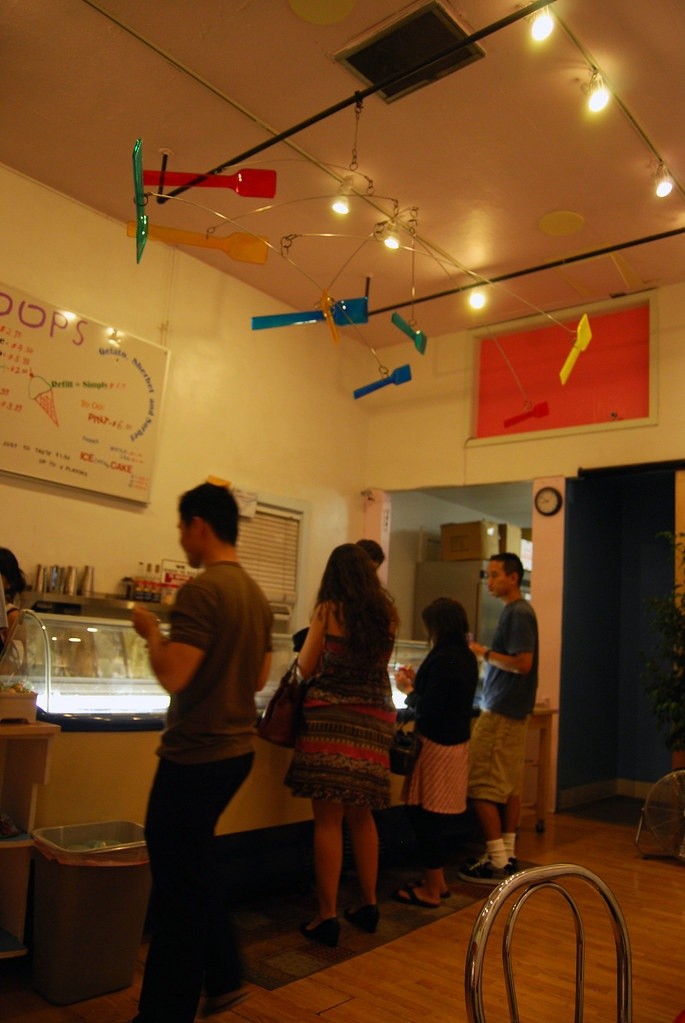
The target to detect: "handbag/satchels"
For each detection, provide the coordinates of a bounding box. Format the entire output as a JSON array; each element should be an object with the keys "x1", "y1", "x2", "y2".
[
  {"x1": 389, "y1": 697, "x2": 421, "y2": 776},
  {"x1": 254, "y1": 658, "x2": 312, "y2": 749}
]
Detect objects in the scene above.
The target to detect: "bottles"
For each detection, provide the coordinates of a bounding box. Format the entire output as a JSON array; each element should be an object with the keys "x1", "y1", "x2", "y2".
[{"x1": 133, "y1": 561, "x2": 163, "y2": 602}]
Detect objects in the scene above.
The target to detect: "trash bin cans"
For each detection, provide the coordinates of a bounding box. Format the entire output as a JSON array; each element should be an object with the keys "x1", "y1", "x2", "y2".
[{"x1": 25, "y1": 817, "x2": 149, "y2": 1005}]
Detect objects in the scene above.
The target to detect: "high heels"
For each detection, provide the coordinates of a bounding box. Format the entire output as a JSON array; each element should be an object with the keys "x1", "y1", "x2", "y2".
[
  {"x1": 299, "y1": 916, "x2": 340, "y2": 948},
  {"x1": 344, "y1": 904, "x2": 380, "y2": 934}
]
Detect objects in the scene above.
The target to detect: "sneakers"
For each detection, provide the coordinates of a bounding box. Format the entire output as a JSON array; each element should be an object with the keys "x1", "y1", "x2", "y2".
[
  {"x1": 503, "y1": 858, "x2": 520, "y2": 876},
  {"x1": 457, "y1": 853, "x2": 507, "y2": 884}
]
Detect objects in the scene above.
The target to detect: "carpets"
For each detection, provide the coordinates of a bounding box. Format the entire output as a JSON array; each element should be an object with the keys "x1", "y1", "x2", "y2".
[{"x1": 231, "y1": 858, "x2": 542, "y2": 993}]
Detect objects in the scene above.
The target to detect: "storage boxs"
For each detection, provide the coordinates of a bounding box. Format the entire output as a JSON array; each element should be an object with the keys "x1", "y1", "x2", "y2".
[
  {"x1": 439, "y1": 520, "x2": 499, "y2": 561},
  {"x1": 520, "y1": 527, "x2": 532, "y2": 539},
  {"x1": 498, "y1": 523, "x2": 522, "y2": 558}
]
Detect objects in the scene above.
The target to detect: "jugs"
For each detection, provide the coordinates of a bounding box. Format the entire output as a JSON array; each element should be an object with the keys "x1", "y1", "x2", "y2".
[{"x1": 118, "y1": 577, "x2": 134, "y2": 599}]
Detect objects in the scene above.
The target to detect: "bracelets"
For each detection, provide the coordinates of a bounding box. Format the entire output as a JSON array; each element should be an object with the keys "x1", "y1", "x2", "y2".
[{"x1": 484, "y1": 647, "x2": 494, "y2": 664}]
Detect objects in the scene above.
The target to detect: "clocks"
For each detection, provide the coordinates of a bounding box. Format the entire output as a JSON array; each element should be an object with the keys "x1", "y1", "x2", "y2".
[{"x1": 533, "y1": 486, "x2": 563, "y2": 518}]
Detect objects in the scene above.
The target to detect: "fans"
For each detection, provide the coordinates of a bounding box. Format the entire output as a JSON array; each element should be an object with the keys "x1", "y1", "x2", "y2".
[{"x1": 634, "y1": 769, "x2": 685, "y2": 862}]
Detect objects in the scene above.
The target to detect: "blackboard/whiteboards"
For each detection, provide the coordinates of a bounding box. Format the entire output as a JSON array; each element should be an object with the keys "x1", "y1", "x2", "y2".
[{"x1": 0, "y1": 280, "x2": 172, "y2": 505}]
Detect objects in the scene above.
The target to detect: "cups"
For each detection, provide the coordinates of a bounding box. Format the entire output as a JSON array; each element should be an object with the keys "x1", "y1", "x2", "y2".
[{"x1": 32, "y1": 564, "x2": 95, "y2": 598}]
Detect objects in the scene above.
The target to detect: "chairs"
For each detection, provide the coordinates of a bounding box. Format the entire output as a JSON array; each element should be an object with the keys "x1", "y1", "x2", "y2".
[{"x1": 463, "y1": 862, "x2": 633, "y2": 1023}]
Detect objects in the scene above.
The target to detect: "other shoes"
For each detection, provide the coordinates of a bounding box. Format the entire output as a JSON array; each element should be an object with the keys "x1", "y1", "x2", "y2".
[{"x1": 200, "y1": 983, "x2": 254, "y2": 1019}]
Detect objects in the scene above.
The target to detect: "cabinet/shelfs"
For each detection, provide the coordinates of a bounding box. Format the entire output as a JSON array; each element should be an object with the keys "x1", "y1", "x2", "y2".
[{"x1": 0, "y1": 720, "x2": 61, "y2": 961}]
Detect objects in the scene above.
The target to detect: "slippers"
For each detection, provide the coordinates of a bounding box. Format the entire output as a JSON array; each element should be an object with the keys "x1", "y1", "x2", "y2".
[
  {"x1": 407, "y1": 880, "x2": 451, "y2": 899},
  {"x1": 394, "y1": 887, "x2": 440, "y2": 908}
]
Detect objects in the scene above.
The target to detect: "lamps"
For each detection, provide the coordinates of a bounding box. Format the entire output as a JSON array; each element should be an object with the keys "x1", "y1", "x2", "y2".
[
  {"x1": 588, "y1": 73, "x2": 612, "y2": 111},
  {"x1": 332, "y1": 175, "x2": 358, "y2": 214},
  {"x1": 531, "y1": 4, "x2": 553, "y2": 41},
  {"x1": 385, "y1": 219, "x2": 404, "y2": 250},
  {"x1": 649, "y1": 163, "x2": 674, "y2": 196}
]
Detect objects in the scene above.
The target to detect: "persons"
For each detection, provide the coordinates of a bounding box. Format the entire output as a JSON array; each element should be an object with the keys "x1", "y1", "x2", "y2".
[
  {"x1": 284, "y1": 539, "x2": 401, "y2": 947},
  {"x1": 456, "y1": 551, "x2": 539, "y2": 887},
  {"x1": 127, "y1": 481, "x2": 275, "y2": 1023},
  {"x1": 0, "y1": 546, "x2": 27, "y2": 673},
  {"x1": 392, "y1": 598, "x2": 480, "y2": 910}
]
